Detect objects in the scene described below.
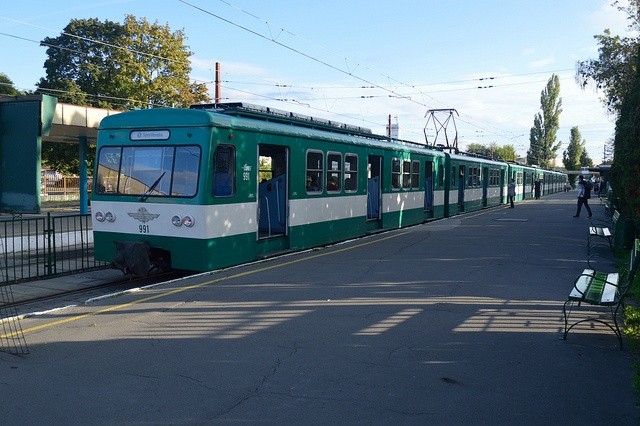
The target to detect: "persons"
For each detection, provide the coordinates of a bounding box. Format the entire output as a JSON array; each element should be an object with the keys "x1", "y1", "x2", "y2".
[
  {"x1": 535, "y1": 177, "x2": 543, "y2": 200},
  {"x1": 508, "y1": 178, "x2": 517, "y2": 206},
  {"x1": 589, "y1": 180, "x2": 607, "y2": 196},
  {"x1": 573, "y1": 175, "x2": 592, "y2": 219}
]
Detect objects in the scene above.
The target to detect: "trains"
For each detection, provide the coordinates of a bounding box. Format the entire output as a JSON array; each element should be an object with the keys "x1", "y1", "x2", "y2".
[{"x1": 90, "y1": 101, "x2": 568, "y2": 278}]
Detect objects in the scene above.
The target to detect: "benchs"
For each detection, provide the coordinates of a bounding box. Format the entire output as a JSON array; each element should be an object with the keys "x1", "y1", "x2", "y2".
[
  {"x1": 561, "y1": 239, "x2": 639, "y2": 349},
  {"x1": 586, "y1": 208, "x2": 623, "y2": 264}
]
